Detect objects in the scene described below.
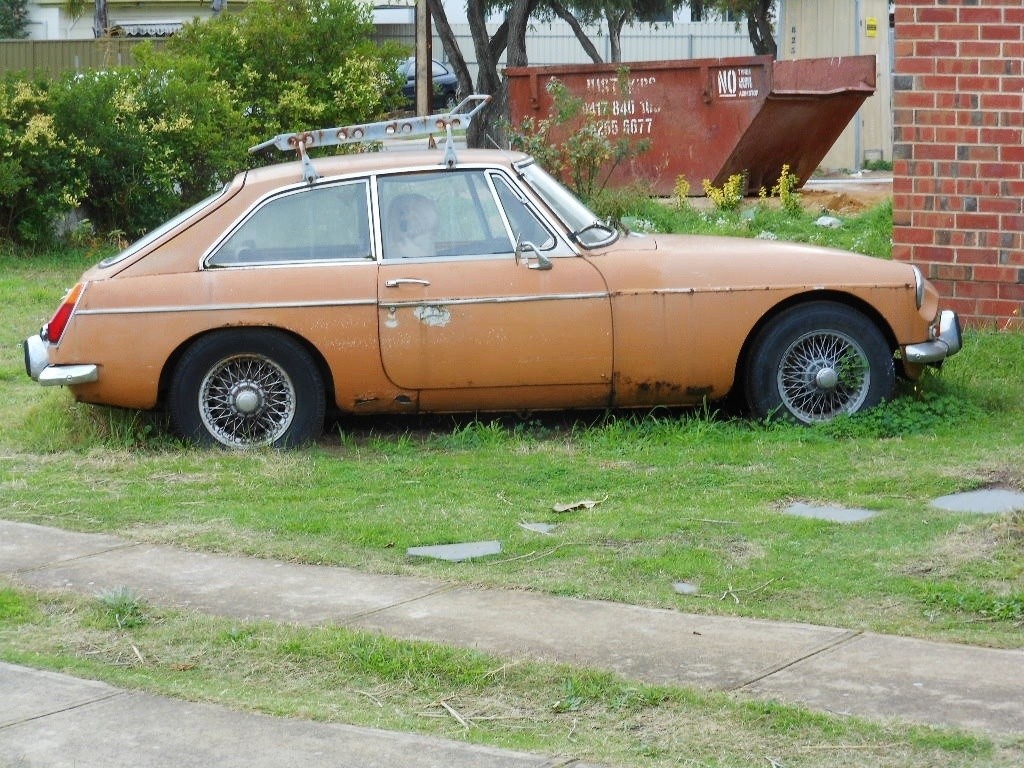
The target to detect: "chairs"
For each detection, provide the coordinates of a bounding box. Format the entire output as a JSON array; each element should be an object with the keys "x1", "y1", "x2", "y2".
[{"x1": 384, "y1": 195, "x2": 442, "y2": 260}]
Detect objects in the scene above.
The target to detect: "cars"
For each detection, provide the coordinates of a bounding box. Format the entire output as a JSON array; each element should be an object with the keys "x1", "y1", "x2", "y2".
[
  {"x1": 23, "y1": 93, "x2": 964, "y2": 452},
  {"x1": 395, "y1": 56, "x2": 463, "y2": 108}
]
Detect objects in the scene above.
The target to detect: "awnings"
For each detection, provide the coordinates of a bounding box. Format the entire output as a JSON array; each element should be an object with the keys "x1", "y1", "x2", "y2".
[{"x1": 112, "y1": 20, "x2": 182, "y2": 36}]
